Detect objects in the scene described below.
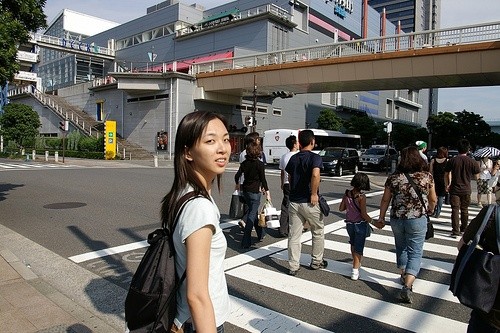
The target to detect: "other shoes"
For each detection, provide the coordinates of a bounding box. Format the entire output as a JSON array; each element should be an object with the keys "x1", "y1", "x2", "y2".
[
  {"x1": 400, "y1": 287, "x2": 414, "y2": 304},
  {"x1": 351, "y1": 271, "x2": 360, "y2": 280},
  {"x1": 289, "y1": 266, "x2": 301, "y2": 275},
  {"x1": 451, "y1": 233, "x2": 460, "y2": 238},
  {"x1": 477, "y1": 202, "x2": 483, "y2": 208},
  {"x1": 399, "y1": 275, "x2": 406, "y2": 285},
  {"x1": 310, "y1": 260, "x2": 328, "y2": 269}
]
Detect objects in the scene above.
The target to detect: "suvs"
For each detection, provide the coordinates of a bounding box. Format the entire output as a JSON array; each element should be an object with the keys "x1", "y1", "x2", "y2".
[
  {"x1": 359, "y1": 147, "x2": 398, "y2": 172},
  {"x1": 317, "y1": 148, "x2": 360, "y2": 177}
]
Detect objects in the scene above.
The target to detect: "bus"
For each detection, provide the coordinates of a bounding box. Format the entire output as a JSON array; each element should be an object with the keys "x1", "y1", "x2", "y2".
[{"x1": 262, "y1": 128, "x2": 361, "y2": 165}]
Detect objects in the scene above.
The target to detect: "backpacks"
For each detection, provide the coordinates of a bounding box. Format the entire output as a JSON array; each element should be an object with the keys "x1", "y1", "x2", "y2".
[{"x1": 125, "y1": 191, "x2": 212, "y2": 333}]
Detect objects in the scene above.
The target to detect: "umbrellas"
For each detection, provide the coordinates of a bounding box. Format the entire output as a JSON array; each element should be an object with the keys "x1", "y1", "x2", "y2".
[{"x1": 473, "y1": 147, "x2": 500, "y2": 157}]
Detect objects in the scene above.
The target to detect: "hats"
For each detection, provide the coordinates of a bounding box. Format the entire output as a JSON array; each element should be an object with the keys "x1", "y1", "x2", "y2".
[{"x1": 415, "y1": 141, "x2": 428, "y2": 150}]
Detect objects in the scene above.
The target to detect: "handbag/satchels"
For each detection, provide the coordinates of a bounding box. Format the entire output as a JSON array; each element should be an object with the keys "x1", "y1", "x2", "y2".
[
  {"x1": 229, "y1": 191, "x2": 244, "y2": 220},
  {"x1": 258, "y1": 202, "x2": 267, "y2": 228},
  {"x1": 448, "y1": 203, "x2": 500, "y2": 313},
  {"x1": 487, "y1": 169, "x2": 500, "y2": 187},
  {"x1": 318, "y1": 196, "x2": 330, "y2": 217},
  {"x1": 264, "y1": 201, "x2": 280, "y2": 229},
  {"x1": 425, "y1": 222, "x2": 434, "y2": 239},
  {"x1": 365, "y1": 222, "x2": 372, "y2": 237}
]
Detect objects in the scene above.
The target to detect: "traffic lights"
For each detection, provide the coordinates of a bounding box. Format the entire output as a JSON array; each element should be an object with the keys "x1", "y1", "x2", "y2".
[
  {"x1": 58, "y1": 120, "x2": 65, "y2": 131},
  {"x1": 245, "y1": 115, "x2": 251, "y2": 126},
  {"x1": 383, "y1": 122, "x2": 389, "y2": 132}
]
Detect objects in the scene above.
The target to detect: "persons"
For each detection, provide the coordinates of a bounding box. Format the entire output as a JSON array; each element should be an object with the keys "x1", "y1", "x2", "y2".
[
  {"x1": 339, "y1": 172, "x2": 383, "y2": 280},
  {"x1": 457, "y1": 170, "x2": 500, "y2": 332},
  {"x1": 476, "y1": 156, "x2": 492, "y2": 208},
  {"x1": 377, "y1": 147, "x2": 437, "y2": 303},
  {"x1": 429, "y1": 147, "x2": 451, "y2": 217},
  {"x1": 490, "y1": 159, "x2": 500, "y2": 200},
  {"x1": 234, "y1": 141, "x2": 270, "y2": 249},
  {"x1": 416, "y1": 141, "x2": 427, "y2": 161},
  {"x1": 444, "y1": 143, "x2": 481, "y2": 235},
  {"x1": 279, "y1": 135, "x2": 308, "y2": 237},
  {"x1": 161, "y1": 111, "x2": 231, "y2": 333},
  {"x1": 239, "y1": 132, "x2": 260, "y2": 234},
  {"x1": 284, "y1": 130, "x2": 328, "y2": 276}
]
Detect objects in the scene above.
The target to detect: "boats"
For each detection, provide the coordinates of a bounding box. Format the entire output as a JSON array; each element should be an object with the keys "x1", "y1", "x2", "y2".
[{"x1": 272, "y1": 90, "x2": 290, "y2": 98}]
